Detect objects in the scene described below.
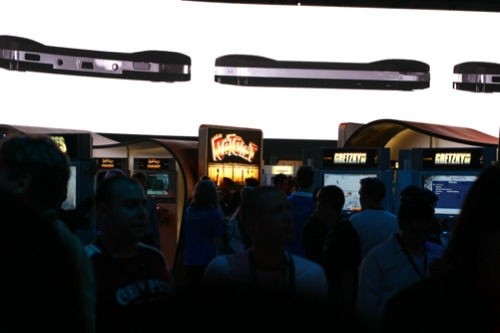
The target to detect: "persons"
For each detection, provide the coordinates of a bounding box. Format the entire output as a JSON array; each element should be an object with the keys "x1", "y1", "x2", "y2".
[{"x1": 0, "y1": 133, "x2": 500, "y2": 333}]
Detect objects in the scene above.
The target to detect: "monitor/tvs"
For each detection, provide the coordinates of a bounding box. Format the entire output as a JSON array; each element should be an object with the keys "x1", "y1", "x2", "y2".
[
  {"x1": 323, "y1": 171, "x2": 378, "y2": 213},
  {"x1": 141, "y1": 173, "x2": 173, "y2": 197},
  {"x1": 421, "y1": 171, "x2": 480, "y2": 219}
]
